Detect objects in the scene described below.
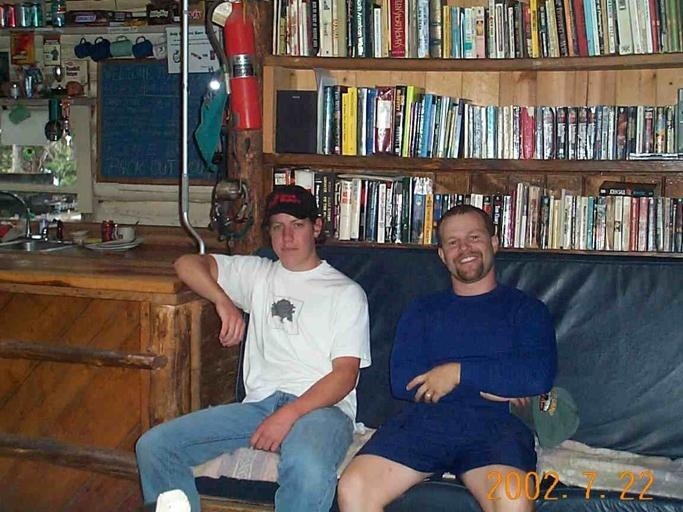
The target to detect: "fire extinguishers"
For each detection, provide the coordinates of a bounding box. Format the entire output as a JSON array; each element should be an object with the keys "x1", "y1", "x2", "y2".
[{"x1": 205, "y1": 0, "x2": 262, "y2": 132}]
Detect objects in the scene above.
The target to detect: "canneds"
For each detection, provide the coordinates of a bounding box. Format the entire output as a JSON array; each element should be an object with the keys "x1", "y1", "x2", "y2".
[
  {"x1": 0, "y1": 3, "x2": 46, "y2": 28},
  {"x1": 51, "y1": 1, "x2": 67, "y2": 27}
]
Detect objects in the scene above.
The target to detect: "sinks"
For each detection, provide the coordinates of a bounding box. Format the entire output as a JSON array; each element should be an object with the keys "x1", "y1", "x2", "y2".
[{"x1": 0, "y1": 236, "x2": 79, "y2": 254}]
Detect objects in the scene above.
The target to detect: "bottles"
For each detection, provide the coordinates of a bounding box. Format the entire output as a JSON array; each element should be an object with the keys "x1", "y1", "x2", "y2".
[{"x1": 30, "y1": 213, "x2": 47, "y2": 235}]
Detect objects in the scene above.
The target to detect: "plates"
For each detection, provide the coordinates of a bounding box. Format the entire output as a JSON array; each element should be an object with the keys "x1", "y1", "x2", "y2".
[{"x1": 87, "y1": 243, "x2": 136, "y2": 252}]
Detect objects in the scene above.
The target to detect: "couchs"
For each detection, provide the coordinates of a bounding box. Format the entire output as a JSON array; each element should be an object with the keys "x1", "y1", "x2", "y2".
[{"x1": 190, "y1": 241, "x2": 682, "y2": 512}]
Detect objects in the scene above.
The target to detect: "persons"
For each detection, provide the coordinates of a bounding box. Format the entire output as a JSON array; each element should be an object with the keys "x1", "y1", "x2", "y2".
[
  {"x1": 337, "y1": 205, "x2": 558, "y2": 512},
  {"x1": 135, "y1": 185, "x2": 372, "y2": 512}
]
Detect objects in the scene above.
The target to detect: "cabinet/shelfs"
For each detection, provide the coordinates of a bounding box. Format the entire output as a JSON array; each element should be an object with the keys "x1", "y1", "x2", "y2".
[
  {"x1": 261, "y1": 51, "x2": 682, "y2": 258},
  {"x1": 0, "y1": 23, "x2": 179, "y2": 107}
]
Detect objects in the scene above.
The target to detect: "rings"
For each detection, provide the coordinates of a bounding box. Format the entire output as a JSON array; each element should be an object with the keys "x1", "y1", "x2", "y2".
[{"x1": 425, "y1": 393, "x2": 432, "y2": 400}]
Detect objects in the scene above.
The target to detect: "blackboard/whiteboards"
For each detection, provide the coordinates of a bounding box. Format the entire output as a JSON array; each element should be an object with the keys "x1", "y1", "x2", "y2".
[{"x1": 96, "y1": 59, "x2": 230, "y2": 186}]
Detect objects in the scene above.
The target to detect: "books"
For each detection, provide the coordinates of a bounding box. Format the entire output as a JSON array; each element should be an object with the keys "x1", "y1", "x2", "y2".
[
  {"x1": 272, "y1": 0, "x2": 682, "y2": 59},
  {"x1": 312, "y1": 67, "x2": 683, "y2": 161},
  {"x1": 277, "y1": 166, "x2": 683, "y2": 254}
]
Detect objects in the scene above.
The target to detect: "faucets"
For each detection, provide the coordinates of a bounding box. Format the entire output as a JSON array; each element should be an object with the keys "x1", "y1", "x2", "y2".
[{"x1": 0, "y1": 189, "x2": 31, "y2": 238}]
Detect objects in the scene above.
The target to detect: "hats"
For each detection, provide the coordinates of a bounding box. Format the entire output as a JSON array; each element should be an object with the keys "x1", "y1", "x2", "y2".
[
  {"x1": 265, "y1": 185, "x2": 316, "y2": 224},
  {"x1": 508, "y1": 385, "x2": 582, "y2": 450}
]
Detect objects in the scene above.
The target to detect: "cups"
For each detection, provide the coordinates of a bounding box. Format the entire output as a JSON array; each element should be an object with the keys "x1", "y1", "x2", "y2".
[
  {"x1": 74, "y1": 36, "x2": 169, "y2": 62},
  {"x1": 115, "y1": 228, "x2": 136, "y2": 242}
]
